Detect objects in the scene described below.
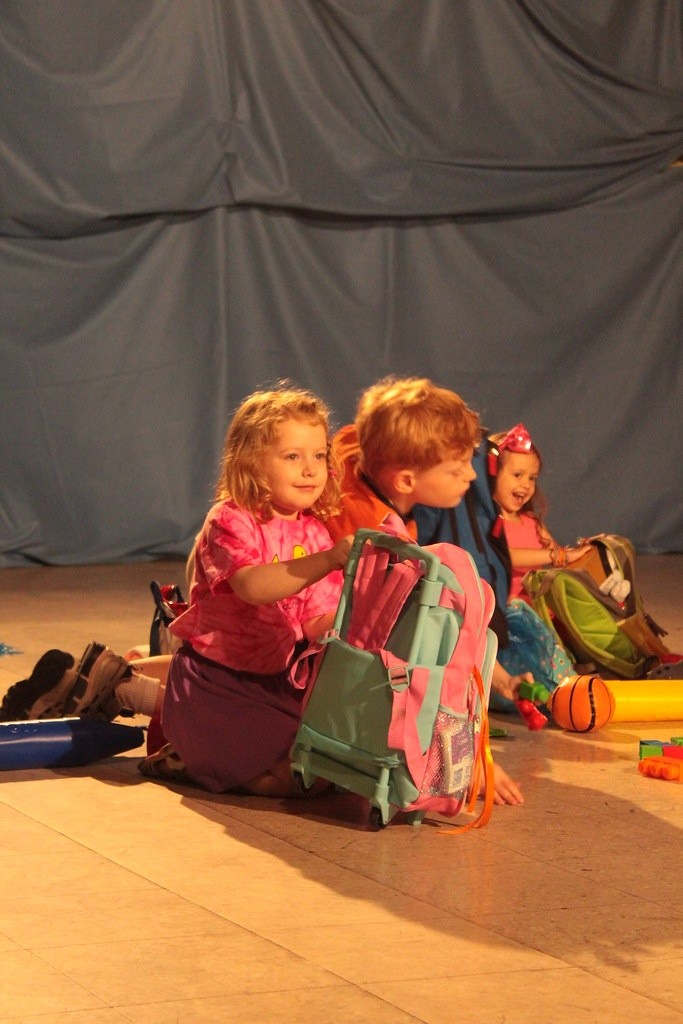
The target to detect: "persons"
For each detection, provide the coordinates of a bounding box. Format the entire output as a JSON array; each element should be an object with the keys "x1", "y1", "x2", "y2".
[
  {"x1": 137, "y1": 390, "x2": 355, "y2": 793},
  {"x1": 485, "y1": 422, "x2": 592, "y2": 620},
  {"x1": 0, "y1": 376, "x2": 535, "y2": 804}
]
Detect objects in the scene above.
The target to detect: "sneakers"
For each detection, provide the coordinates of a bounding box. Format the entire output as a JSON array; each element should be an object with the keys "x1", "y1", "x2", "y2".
[
  {"x1": 64, "y1": 641, "x2": 133, "y2": 722},
  {"x1": 0, "y1": 649, "x2": 80, "y2": 721}
]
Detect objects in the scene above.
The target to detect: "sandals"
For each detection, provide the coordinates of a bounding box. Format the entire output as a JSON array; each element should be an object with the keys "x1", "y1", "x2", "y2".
[{"x1": 139, "y1": 742, "x2": 185, "y2": 777}]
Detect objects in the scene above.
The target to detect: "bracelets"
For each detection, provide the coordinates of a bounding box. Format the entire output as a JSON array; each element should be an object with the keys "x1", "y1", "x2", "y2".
[{"x1": 553, "y1": 546, "x2": 566, "y2": 567}]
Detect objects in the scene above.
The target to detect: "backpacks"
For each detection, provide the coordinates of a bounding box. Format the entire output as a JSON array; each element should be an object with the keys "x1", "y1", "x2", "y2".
[
  {"x1": 518, "y1": 531, "x2": 669, "y2": 680},
  {"x1": 488, "y1": 599, "x2": 578, "y2": 712}
]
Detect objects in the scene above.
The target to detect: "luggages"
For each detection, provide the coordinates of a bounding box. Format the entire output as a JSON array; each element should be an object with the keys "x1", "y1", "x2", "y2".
[{"x1": 288, "y1": 529, "x2": 499, "y2": 828}]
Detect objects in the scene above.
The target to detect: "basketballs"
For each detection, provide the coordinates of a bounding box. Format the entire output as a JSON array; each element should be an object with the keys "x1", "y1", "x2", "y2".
[{"x1": 549, "y1": 674, "x2": 616, "y2": 733}]
